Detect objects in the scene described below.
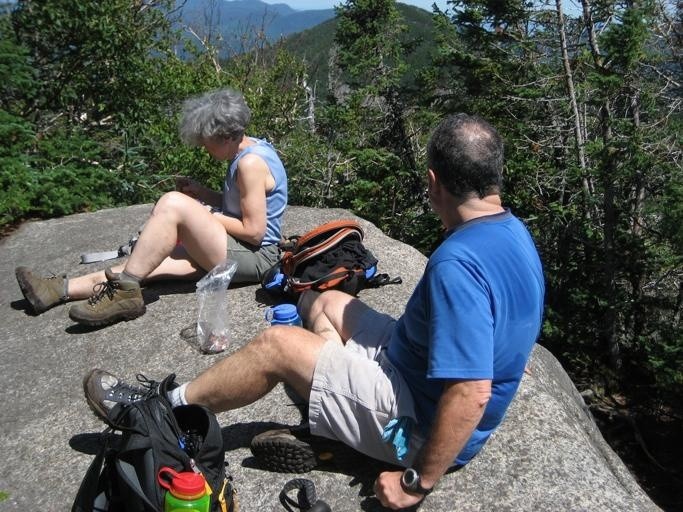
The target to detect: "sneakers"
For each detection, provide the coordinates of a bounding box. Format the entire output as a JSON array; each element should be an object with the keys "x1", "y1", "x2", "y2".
[
  {"x1": 14, "y1": 266, "x2": 69, "y2": 315},
  {"x1": 68, "y1": 267, "x2": 146, "y2": 327},
  {"x1": 83, "y1": 368, "x2": 181, "y2": 436}
]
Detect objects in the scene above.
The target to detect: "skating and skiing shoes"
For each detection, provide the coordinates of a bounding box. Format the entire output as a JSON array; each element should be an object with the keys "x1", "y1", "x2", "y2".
[{"x1": 250, "y1": 422, "x2": 347, "y2": 474}]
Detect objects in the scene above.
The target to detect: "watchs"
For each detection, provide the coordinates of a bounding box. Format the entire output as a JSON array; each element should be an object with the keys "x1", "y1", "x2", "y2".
[{"x1": 400, "y1": 465, "x2": 434, "y2": 496}]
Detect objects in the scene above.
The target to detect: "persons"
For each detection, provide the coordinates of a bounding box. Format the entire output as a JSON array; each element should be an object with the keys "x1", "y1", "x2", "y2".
[
  {"x1": 82, "y1": 111, "x2": 547, "y2": 511},
  {"x1": 13, "y1": 87, "x2": 289, "y2": 329}
]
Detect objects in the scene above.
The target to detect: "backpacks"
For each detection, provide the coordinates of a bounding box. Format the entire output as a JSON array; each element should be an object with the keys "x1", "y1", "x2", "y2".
[
  {"x1": 69, "y1": 394, "x2": 236, "y2": 512},
  {"x1": 259, "y1": 218, "x2": 405, "y2": 310}
]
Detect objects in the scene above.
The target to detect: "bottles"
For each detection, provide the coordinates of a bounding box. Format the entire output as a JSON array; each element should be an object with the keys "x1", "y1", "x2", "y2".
[
  {"x1": 158, "y1": 468, "x2": 209, "y2": 512},
  {"x1": 266, "y1": 305, "x2": 302, "y2": 328}
]
